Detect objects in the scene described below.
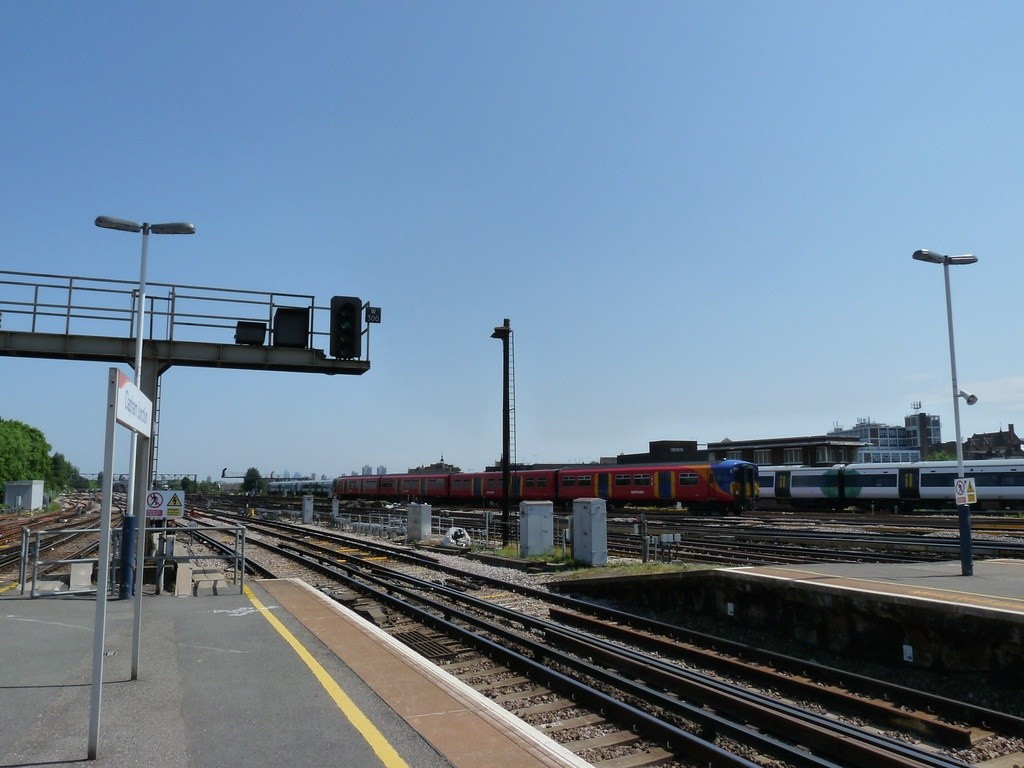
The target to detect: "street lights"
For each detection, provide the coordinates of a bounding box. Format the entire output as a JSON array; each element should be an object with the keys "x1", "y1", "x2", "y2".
[
  {"x1": 93, "y1": 215, "x2": 196, "y2": 600},
  {"x1": 490, "y1": 318, "x2": 512, "y2": 546},
  {"x1": 912, "y1": 249, "x2": 980, "y2": 576}
]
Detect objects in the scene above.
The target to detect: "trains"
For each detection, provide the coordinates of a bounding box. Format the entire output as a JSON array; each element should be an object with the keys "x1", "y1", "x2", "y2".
[
  {"x1": 266, "y1": 479, "x2": 333, "y2": 497},
  {"x1": 332, "y1": 458, "x2": 761, "y2": 517},
  {"x1": 760, "y1": 459, "x2": 1024, "y2": 511}
]
